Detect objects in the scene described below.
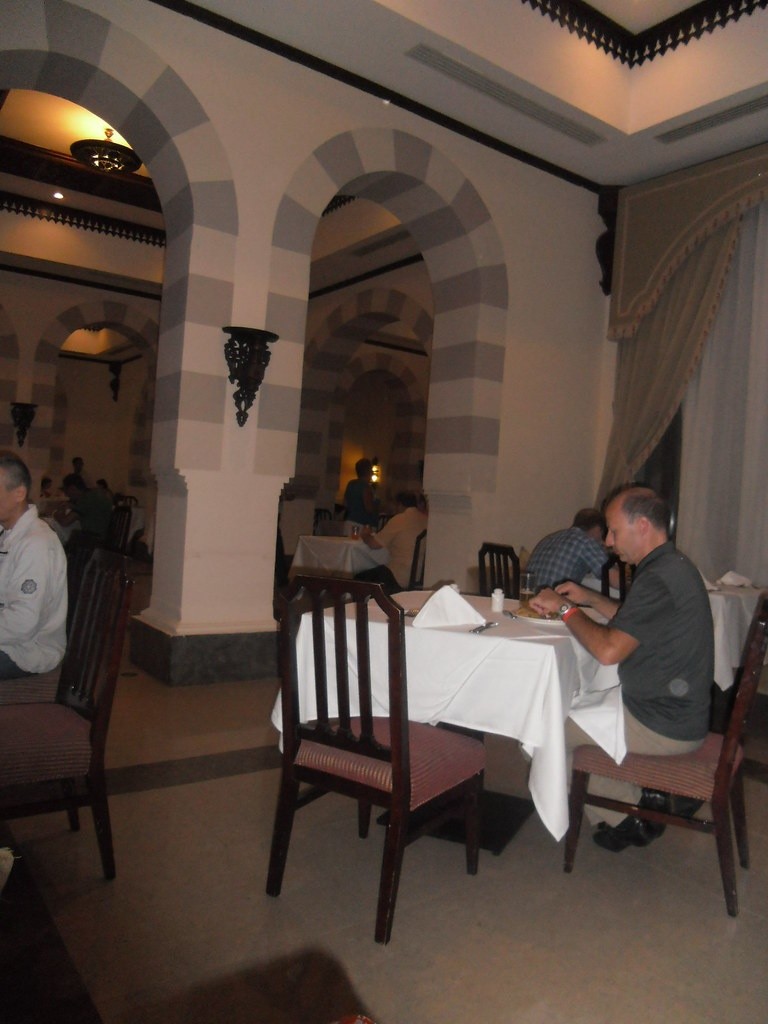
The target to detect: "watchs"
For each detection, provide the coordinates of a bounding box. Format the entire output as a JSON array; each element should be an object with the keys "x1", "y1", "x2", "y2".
[{"x1": 558, "y1": 603, "x2": 577, "y2": 615}]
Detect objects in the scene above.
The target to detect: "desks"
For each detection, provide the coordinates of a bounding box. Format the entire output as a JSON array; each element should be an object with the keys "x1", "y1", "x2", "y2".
[
  {"x1": 39, "y1": 494, "x2": 146, "y2": 545},
  {"x1": 287, "y1": 535, "x2": 390, "y2": 586},
  {"x1": 707, "y1": 584, "x2": 768, "y2": 693}
]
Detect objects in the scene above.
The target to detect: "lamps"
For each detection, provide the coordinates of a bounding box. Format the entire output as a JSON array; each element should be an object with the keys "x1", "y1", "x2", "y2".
[{"x1": 70, "y1": 127, "x2": 142, "y2": 176}]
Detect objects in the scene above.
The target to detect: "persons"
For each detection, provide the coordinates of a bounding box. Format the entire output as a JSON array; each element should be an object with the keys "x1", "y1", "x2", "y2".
[
  {"x1": 342, "y1": 458, "x2": 429, "y2": 602},
  {"x1": 0, "y1": 447, "x2": 67, "y2": 680},
  {"x1": 529, "y1": 483, "x2": 714, "y2": 851},
  {"x1": 41, "y1": 456, "x2": 113, "y2": 537},
  {"x1": 526, "y1": 507, "x2": 628, "y2": 598}
]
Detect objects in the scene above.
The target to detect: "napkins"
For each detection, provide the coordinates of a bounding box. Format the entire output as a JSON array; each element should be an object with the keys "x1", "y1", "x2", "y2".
[
  {"x1": 412, "y1": 585, "x2": 486, "y2": 628},
  {"x1": 716, "y1": 571, "x2": 752, "y2": 586}
]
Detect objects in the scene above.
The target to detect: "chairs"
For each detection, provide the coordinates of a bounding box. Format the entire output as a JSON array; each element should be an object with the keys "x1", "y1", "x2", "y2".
[
  {"x1": 478, "y1": 542, "x2": 520, "y2": 601},
  {"x1": 563, "y1": 591, "x2": 768, "y2": 916},
  {"x1": 107, "y1": 506, "x2": 132, "y2": 554},
  {"x1": 601, "y1": 552, "x2": 637, "y2": 603},
  {"x1": 0, "y1": 529, "x2": 103, "y2": 706},
  {"x1": 265, "y1": 573, "x2": 487, "y2": 945},
  {"x1": 313, "y1": 508, "x2": 332, "y2": 535},
  {"x1": 0, "y1": 550, "x2": 135, "y2": 880}
]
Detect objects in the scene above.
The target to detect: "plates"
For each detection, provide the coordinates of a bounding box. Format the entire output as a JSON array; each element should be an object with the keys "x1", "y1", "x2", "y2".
[{"x1": 510, "y1": 609, "x2": 565, "y2": 626}]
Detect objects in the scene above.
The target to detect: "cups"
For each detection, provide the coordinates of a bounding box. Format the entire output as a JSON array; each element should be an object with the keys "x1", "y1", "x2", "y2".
[
  {"x1": 364, "y1": 524, "x2": 370, "y2": 535},
  {"x1": 520, "y1": 573, "x2": 536, "y2": 608}
]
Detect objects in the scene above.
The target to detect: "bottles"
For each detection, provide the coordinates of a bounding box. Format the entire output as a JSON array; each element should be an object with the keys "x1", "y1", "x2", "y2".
[
  {"x1": 491, "y1": 588, "x2": 504, "y2": 613},
  {"x1": 352, "y1": 526, "x2": 359, "y2": 539}
]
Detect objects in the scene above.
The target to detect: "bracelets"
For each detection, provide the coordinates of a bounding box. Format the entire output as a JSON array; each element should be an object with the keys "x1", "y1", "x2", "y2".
[{"x1": 562, "y1": 607, "x2": 580, "y2": 622}]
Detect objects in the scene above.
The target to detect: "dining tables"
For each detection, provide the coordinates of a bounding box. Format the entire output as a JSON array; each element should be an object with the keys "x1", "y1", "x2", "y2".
[{"x1": 271, "y1": 590, "x2": 628, "y2": 856}]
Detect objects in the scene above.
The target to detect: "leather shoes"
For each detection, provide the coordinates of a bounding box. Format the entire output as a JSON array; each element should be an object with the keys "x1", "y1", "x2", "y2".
[
  {"x1": 636, "y1": 787, "x2": 669, "y2": 829},
  {"x1": 594, "y1": 814, "x2": 655, "y2": 852}
]
